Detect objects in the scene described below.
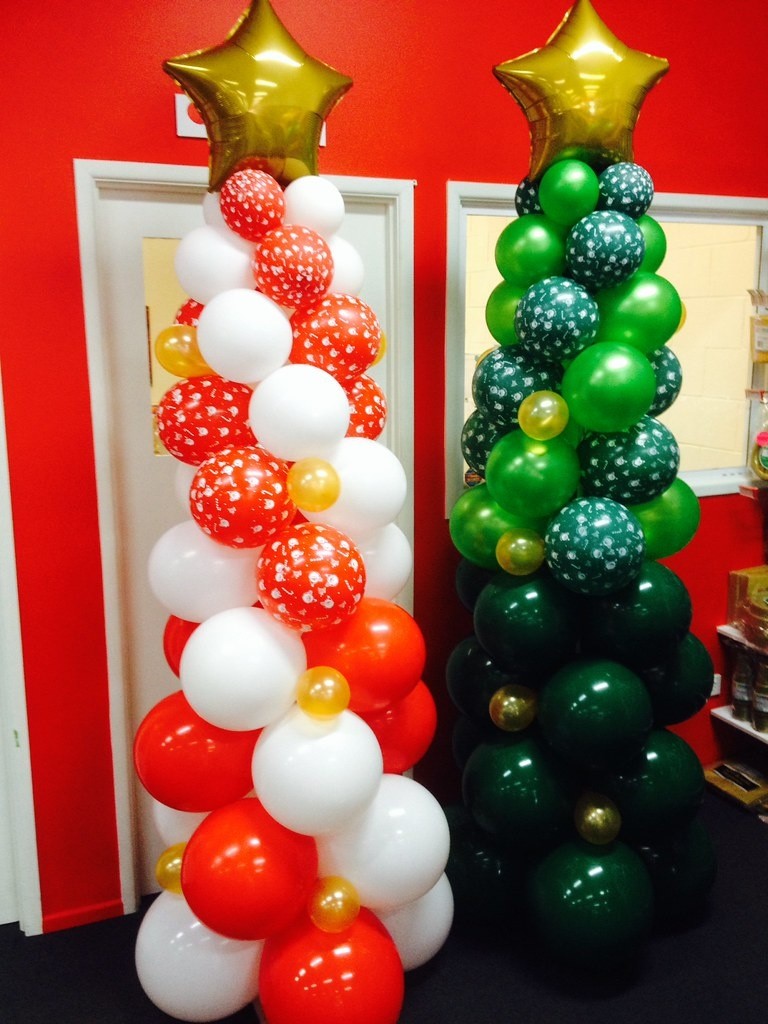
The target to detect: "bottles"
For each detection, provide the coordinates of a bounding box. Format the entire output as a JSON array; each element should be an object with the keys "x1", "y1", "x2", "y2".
[
  {"x1": 730, "y1": 648, "x2": 753, "y2": 721},
  {"x1": 751, "y1": 657, "x2": 768, "y2": 733}
]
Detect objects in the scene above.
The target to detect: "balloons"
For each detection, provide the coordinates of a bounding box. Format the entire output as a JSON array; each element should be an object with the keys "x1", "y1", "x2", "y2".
[{"x1": 132, "y1": 1, "x2": 718, "y2": 1021}]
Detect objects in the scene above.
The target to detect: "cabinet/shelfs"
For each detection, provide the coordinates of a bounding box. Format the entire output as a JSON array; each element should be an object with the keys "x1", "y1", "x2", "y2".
[{"x1": 712, "y1": 624, "x2": 768, "y2": 746}]
